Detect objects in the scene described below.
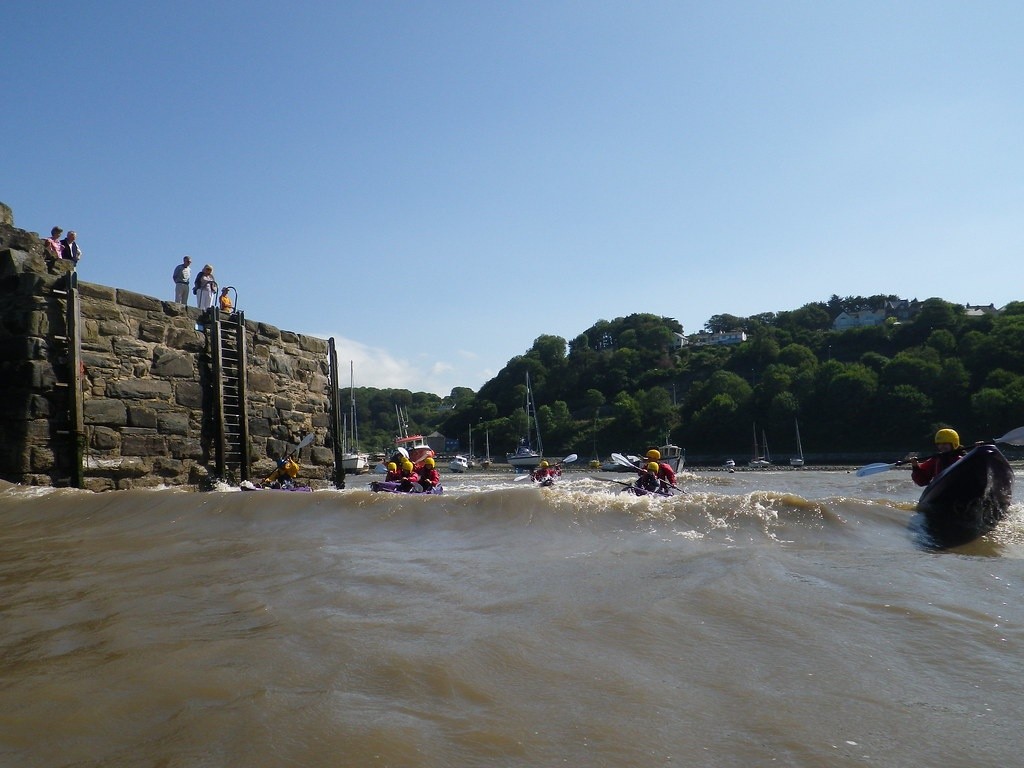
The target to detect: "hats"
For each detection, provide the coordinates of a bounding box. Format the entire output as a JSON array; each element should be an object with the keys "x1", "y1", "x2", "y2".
[{"x1": 221, "y1": 287, "x2": 229, "y2": 291}]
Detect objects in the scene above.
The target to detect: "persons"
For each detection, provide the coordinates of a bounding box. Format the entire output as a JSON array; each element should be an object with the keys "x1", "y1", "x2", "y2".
[
  {"x1": 195, "y1": 264, "x2": 217, "y2": 311},
  {"x1": 531, "y1": 461, "x2": 562, "y2": 483},
  {"x1": 173, "y1": 256, "x2": 192, "y2": 305},
  {"x1": 385, "y1": 457, "x2": 440, "y2": 494},
  {"x1": 910, "y1": 429, "x2": 967, "y2": 488},
  {"x1": 60, "y1": 231, "x2": 82, "y2": 271},
  {"x1": 219, "y1": 286, "x2": 233, "y2": 313},
  {"x1": 638, "y1": 450, "x2": 678, "y2": 489},
  {"x1": 262, "y1": 457, "x2": 299, "y2": 486},
  {"x1": 635, "y1": 462, "x2": 670, "y2": 496},
  {"x1": 43, "y1": 226, "x2": 65, "y2": 260}
]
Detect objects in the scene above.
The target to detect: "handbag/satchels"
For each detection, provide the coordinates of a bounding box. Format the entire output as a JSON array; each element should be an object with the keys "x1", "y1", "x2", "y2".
[{"x1": 192, "y1": 287, "x2": 197, "y2": 295}]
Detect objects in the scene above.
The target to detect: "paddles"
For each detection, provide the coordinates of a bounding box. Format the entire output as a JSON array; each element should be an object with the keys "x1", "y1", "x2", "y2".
[
  {"x1": 375, "y1": 463, "x2": 423, "y2": 492},
  {"x1": 856, "y1": 426, "x2": 1024, "y2": 477},
  {"x1": 590, "y1": 475, "x2": 674, "y2": 497},
  {"x1": 398, "y1": 447, "x2": 443, "y2": 495},
  {"x1": 611, "y1": 453, "x2": 687, "y2": 494},
  {"x1": 260, "y1": 432, "x2": 314, "y2": 484},
  {"x1": 514, "y1": 454, "x2": 577, "y2": 482}
]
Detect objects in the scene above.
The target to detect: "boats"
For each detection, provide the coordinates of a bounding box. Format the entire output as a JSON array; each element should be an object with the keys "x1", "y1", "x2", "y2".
[
  {"x1": 449, "y1": 455, "x2": 469, "y2": 473},
  {"x1": 240, "y1": 482, "x2": 314, "y2": 492},
  {"x1": 599, "y1": 450, "x2": 685, "y2": 474},
  {"x1": 913, "y1": 444, "x2": 1017, "y2": 521},
  {"x1": 528, "y1": 475, "x2": 559, "y2": 486},
  {"x1": 630, "y1": 485, "x2": 674, "y2": 498},
  {"x1": 506, "y1": 452, "x2": 565, "y2": 468},
  {"x1": 726, "y1": 459, "x2": 735, "y2": 467},
  {"x1": 369, "y1": 480, "x2": 444, "y2": 495},
  {"x1": 790, "y1": 459, "x2": 804, "y2": 465},
  {"x1": 747, "y1": 461, "x2": 770, "y2": 468}
]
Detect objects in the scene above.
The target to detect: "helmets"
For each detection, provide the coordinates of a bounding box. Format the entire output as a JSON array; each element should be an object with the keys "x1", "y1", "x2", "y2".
[
  {"x1": 425, "y1": 457, "x2": 435, "y2": 468},
  {"x1": 276, "y1": 459, "x2": 288, "y2": 468},
  {"x1": 647, "y1": 462, "x2": 659, "y2": 474},
  {"x1": 388, "y1": 463, "x2": 397, "y2": 473},
  {"x1": 646, "y1": 449, "x2": 661, "y2": 460},
  {"x1": 401, "y1": 457, "x2": 409, "y2": 463},
  {"x1": 402, "y1": 461, "x2": 413, "y2": 474},
  {"x1": 935, "y1": 429, "x2": 959, "y2": 450},
  {"x1": 541, "y1": 461, "x2": 548, "y2": 467}
]
